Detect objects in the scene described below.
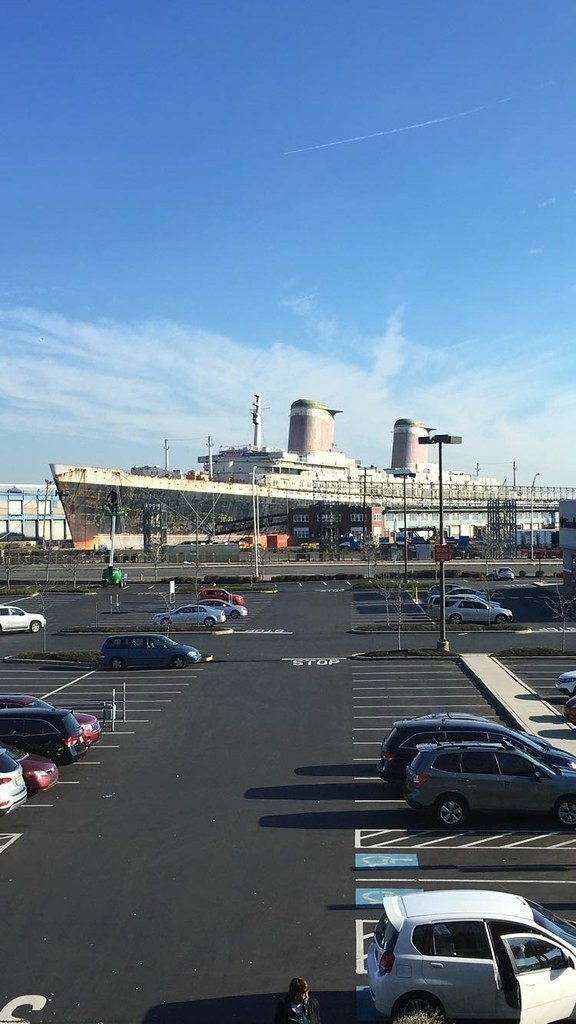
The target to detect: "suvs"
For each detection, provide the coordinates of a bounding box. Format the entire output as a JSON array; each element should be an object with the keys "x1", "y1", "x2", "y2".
[
  {"x1": 0, "y1": 695, "x2": 102, "y2": 747},
  {"x1": 403, "y1": 737, "x2": 575, "y2": 828},
  {"x1": 0, "y1": 605, "x2": 46, "y2": 635},
  {"x1": 0, "y1": 707, "x2": 89, "y2": 767},
  {"x1": 198, "y1": 588, "x2": 245, "y2": 605},
  {"x1": 376, "y1": 717, "x2": 576, "y2": 785}
]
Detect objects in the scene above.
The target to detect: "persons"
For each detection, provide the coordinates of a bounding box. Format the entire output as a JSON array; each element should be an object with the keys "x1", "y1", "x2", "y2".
[
  {"x1": 275, "y1": 977, "x2": 321, "y2": 1024},
  {"x1": 505, "y1": 939, "x2": 533, "y2": 1010}
]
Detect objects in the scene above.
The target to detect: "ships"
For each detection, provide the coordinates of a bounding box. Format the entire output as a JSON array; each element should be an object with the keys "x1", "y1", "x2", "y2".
[{"x1": 48, "y1": 392, "x2": 561, "y2": 551}]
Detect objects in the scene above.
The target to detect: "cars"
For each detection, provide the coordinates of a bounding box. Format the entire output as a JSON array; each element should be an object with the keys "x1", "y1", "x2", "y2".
[
  {"x1": 426, "y1": 584, "x2": 514, "y2": 623},
  {"x1": 1, "y1": 741, "x2": 59, "y2": 798},
  {"x1": 153, "y1": 604, "x2": 226, "y2": 627},
  {"x1": 0, "y1": 749, "x2": 28, "y2": 819},
  {"x1": 492, "y1": 567, "x2": 515, "y2": 581},
  {"x1": 192, "y1": 598, "x2": 248, "y2": 619},
  {"x1": 366, "y1": 888, "x2": 576, "y2": 1024},
  {"x1": 555, "y1": 670, "x2": 576, "y2": 725}
]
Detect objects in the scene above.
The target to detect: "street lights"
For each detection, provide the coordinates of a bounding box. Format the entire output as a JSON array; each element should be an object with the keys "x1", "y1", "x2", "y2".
[{"x1": 416, "y1": 434, "x2": 463, "y2": 653}]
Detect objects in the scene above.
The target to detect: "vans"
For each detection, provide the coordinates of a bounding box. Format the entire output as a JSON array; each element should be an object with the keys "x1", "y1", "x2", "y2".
[{"x1": 101, "y1": 634, "x2": 202, "y2": 669}]
